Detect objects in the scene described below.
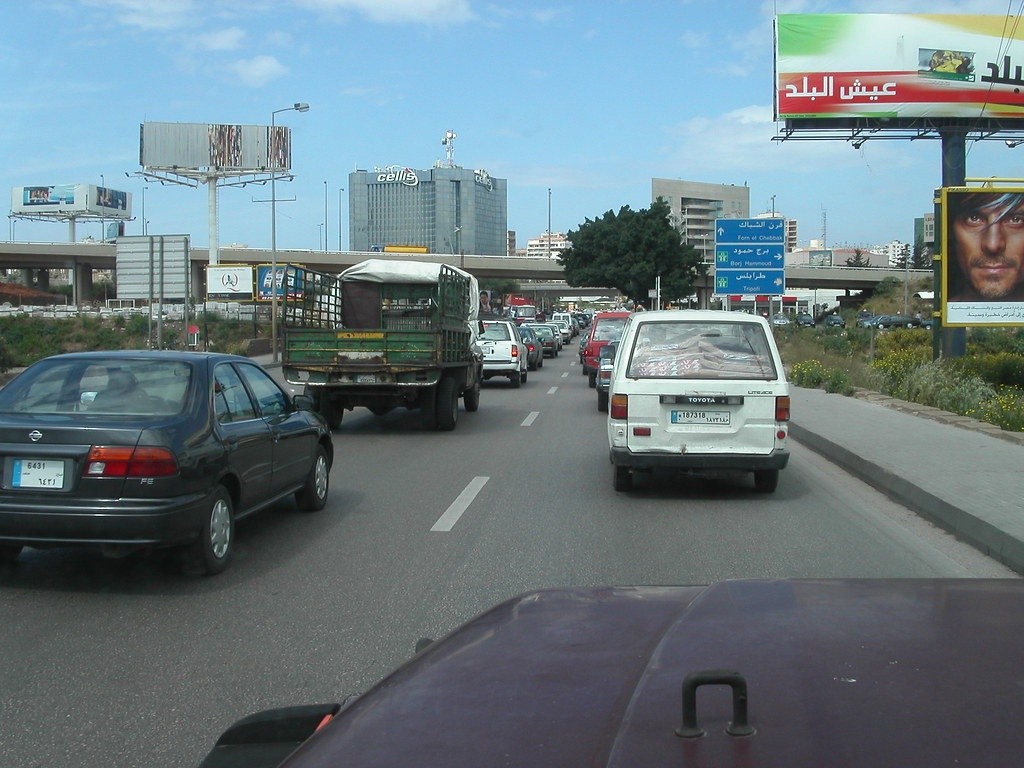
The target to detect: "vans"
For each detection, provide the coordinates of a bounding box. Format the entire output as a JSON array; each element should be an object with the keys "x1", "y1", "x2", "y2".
[{"x1": 597, "y1": 309, "x2": 790, "y2": 492}]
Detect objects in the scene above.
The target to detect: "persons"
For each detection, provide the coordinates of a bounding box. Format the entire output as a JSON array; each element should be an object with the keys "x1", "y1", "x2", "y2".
[
  {"x1": 948, "y1": 193, "x2": 1024, "y2": 302},
  {"x1": 916, "y1": 311, "x2": 923, "y2": 329},
  {"x1": 640, "y1": 325, "x2": 666, "y2": 348},
  {"x1": 480, "y1": 291, "x2": 491, "y2": 312}
]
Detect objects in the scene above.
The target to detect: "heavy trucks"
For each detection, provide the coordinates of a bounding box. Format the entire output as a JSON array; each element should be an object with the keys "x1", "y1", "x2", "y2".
[{"x1": 370, "y1": 245, "x2": 430, "y2": 253}]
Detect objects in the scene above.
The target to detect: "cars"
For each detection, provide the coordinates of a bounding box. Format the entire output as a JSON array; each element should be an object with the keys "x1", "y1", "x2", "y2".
[
  {"x1": 773, "y1": 314, "x2": 790, "y2": 327},
  {"x1": 826, "y1": 315, "x2": 845, "y2": 328},
  {"x1": 578, "y1": 312, "x2": 634, "y2": 412},
  {"x1": 516, "y1": 323, "x2": 563, "y2": 370},
  {"x1": 854, "y1": 312, "x2": 921, "y2": 330},
  {"x1": 0, "y1": 349, "x2": 334, "y2": 574},
  {"x1": 921, "y1": 317, "x2": 933, "y2": 330},
  {"x1": 795, "y1": 315, "x2": 815, "y2": 328},
  {"x1": 545, "y1": 313, "x2": 589, "y2": 345},
  {"x1": 81, "y1": 302, "x2": 260, "y2": 319},
  {"x1": 0, "y1": 305, "x2": 79, "y2": 316}
]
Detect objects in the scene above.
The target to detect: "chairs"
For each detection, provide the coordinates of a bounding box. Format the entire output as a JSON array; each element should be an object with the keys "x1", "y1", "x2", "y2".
[
  {"x1": 92, "y1": 387, "x2": 150, "y2": 412},
  {"x1": 599, "y1": 328, "x2": 616, "y2": 340}
]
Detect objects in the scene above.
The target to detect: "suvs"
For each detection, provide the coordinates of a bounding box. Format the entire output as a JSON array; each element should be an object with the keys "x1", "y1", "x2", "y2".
[{"x1": 474, "y1": 319, "x2": 528, "y2": 388}]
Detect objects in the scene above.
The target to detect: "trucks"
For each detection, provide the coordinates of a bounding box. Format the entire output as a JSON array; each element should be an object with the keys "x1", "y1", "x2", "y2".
[
  {"x1": 280, "y1": 262, "x2": 486, "y2": 430},
  {"x1": 506, "y1": 305, "x2": 545, "y2": 326}
]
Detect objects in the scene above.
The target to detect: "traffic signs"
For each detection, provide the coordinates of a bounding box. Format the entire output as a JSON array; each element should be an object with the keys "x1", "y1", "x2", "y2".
[{"x1": 715, "y1": 217, "x2": 785, "y2": 297}]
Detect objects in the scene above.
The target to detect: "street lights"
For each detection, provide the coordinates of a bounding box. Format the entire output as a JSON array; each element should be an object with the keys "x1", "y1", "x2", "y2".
[
  {"x1": 101, "y1": 175, "x2": 149, "y2": 242},
  {"x1": 339, "y1": 189, "x2": 344, "y2": 252},
  {"x1": 8, "y1": 216, "x2": 16, "y2": 242},
  {"x1": 272, "y1": 102, "x2": 309, "y2": 362},
  {"x1": 318, "y1": 224, "x2": 324, "y2": 250},
  {"x1": 324, "y1": 181, "x2": 328, "y2": 252}
]
